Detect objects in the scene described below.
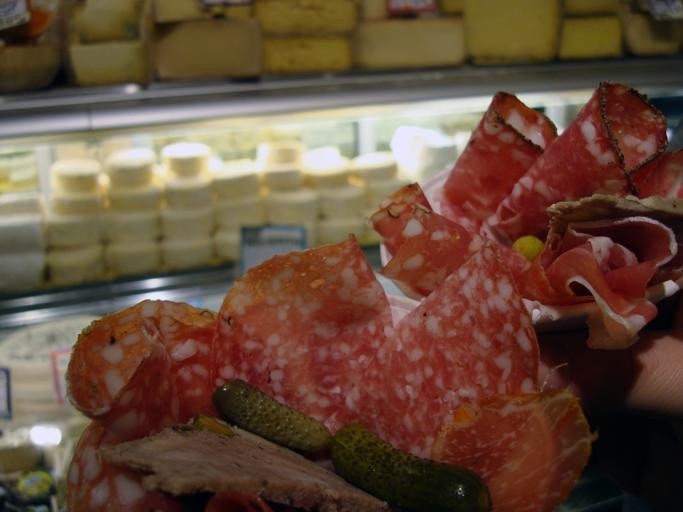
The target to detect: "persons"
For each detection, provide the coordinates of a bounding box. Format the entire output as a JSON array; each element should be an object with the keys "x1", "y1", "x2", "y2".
[{"x1": 537, "y1": 289, "x2": 683, "y2": 415}]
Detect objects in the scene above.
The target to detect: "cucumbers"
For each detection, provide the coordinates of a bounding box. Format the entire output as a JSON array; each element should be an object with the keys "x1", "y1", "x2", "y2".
[
  {"x1": 214, "y1": 380, "x2": 330, "y2": 453},
  {"x1": 328, "y1": 424, "x2": 492, "y2": 512}
]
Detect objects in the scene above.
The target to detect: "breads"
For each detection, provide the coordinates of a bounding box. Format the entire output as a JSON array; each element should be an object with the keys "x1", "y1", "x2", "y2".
[
  {"x1": 67, "y1": 37, "x2": 147, "y2": 84},
  {"x1": 263, "y1": 37, "x2": 351, "y2": 72},
  {"x1": 152, "y1": 21, "x2": 261, "y2": 78},
  {"x1": 463, "y1": 2, "x2": 559, "y2": 64},
  {"x1": 358, "y1": 19, "x2": 465, "y2": 67},
  {"x1": 70, "y1": 4, "x2": 142, "y2": 38},
  {"x1": 565, "y1": 2, "x2": 630, "y2": 17},
  {"x1": 359, "y1": 1, "x2": 463, "y2": 19},
  {"x1": 625, "y1": 15, "x2": 674, "y2": 55},
  {"x1": 153, "y1": 2, "x2": 252, "y2": 21},
  {"x1": 2, "y1": 43, "x2": 58, "y2": 90},
  {"x1": 253, "y1": 2, "x2": 358, "y2": 35},
  {"x1": 558, "y1": 16, "x2": 622, "y2": 57}
]
